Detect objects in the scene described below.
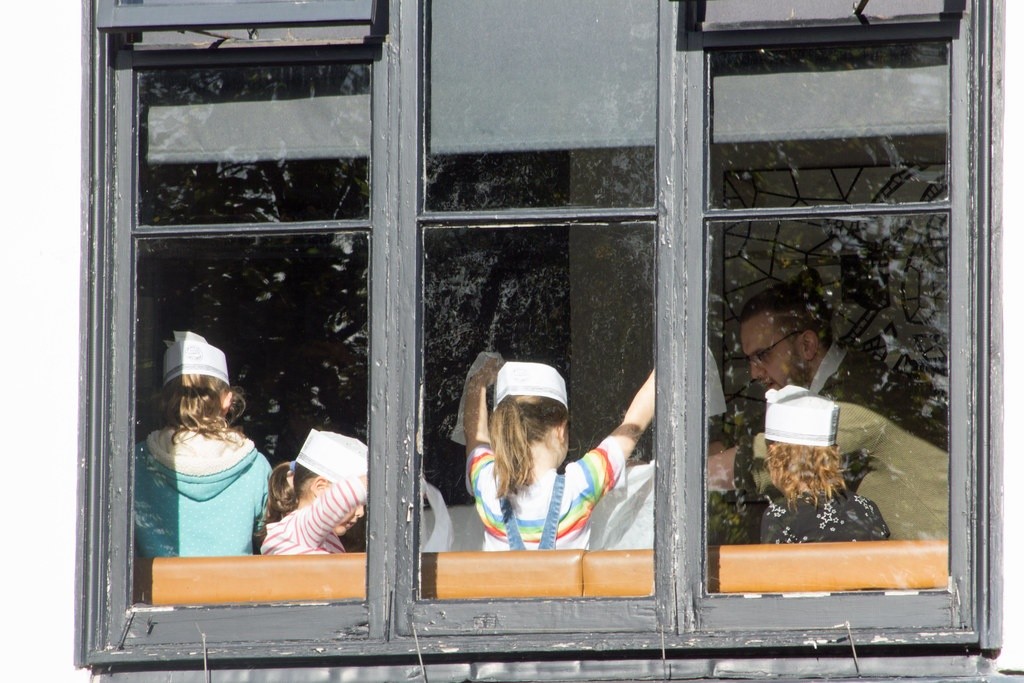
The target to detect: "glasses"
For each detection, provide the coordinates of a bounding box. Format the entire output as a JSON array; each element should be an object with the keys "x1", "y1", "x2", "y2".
[{"x1": 743, "y1": 330, "x2": 803, "y2": 373}]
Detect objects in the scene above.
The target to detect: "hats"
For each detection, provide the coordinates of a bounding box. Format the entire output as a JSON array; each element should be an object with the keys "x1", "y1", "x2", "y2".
[
  {"x1": 493, "y1": 362, "x2": 568, "y2": 414},
  {"x1": 765, "y1": 385, "x2": 840, "y2": 446},
  {"x1": 296, "y1": 428, "x2": 368, "y2": 483},
  {"x1": 161, "y1": 331, "x2": 230, "y2": 390}
]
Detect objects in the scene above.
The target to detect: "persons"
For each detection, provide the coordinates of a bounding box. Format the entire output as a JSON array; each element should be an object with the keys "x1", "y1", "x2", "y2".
[
  {"x1": 256, "y1": 429, "x2": 368, "y2": 555},
  {"x1": 133, "y1": 330, "x2": 274, "y2": 559},
  {"x1": 462, "y1": 358, "x2": 655, "y2": 551},
  {"x1": 760, "y1": 384, "x2": 891, "y2": 543},
  {"x1": 708, "y1": 285, "x2": 949, "y2": 541}
]
